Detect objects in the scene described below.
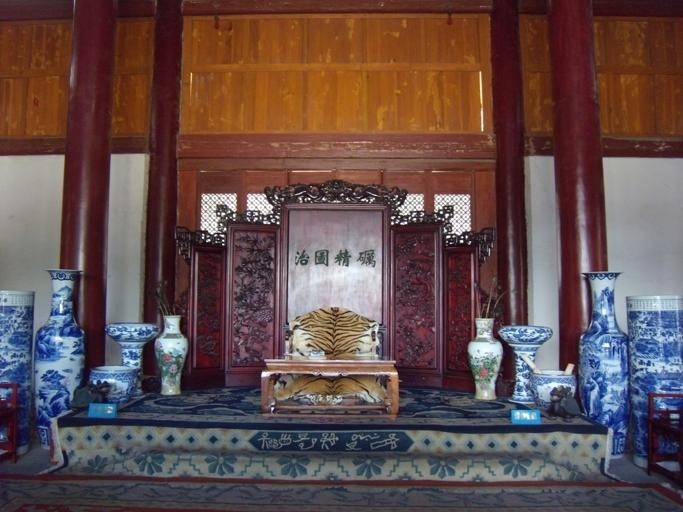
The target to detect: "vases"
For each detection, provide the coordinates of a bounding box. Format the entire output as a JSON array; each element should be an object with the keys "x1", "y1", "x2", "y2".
[
  {"x1": 104, "y1": 323, "x2": 161, "y2": 399},
  {"x1": 154, "y1": 315, "x2": 189, "y2": 396},
  {"x1": 498, "y1": 325, "x2": 553, "y2": 405},
  {"x1": 1, "y1": 290, "x2": 35, "y2": 456},
  {"x1": 626, "y1": 295, "x2": 683, "y2": 470},
  {"x1": 530, "y1": 370, "x2": 577, "y2": 409},
  {"x1": 578, "y1": 271, "x2": 628, "y2": 459},
  {"x1": 467, "y1": 318, "x2": 503, "y2": 401},
  {"x1": 88, "y1": 366, "x2": 138, "y2": 404},
  {"x1": 33, "y1": 268, "x2": 86, "y2": 450}
]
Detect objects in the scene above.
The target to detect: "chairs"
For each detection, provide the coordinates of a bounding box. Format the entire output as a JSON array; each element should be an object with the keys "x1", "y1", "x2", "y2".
[{"x1": 285, "y1": 307, "x2": 380, "y2": 361}]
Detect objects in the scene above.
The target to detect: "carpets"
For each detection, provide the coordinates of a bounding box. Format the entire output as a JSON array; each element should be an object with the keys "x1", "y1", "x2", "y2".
[
  {"x1": 0, "y1": 476, "x2": 683, "y2": 511},
  {"x1": 36, "y1": 388, "x2": 627, "y2": 482}
]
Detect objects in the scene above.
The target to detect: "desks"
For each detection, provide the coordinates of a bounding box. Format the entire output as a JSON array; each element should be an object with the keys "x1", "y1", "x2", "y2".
[{"x1": 261, "y1": 358, "x2": 399, "y2": 414}]
